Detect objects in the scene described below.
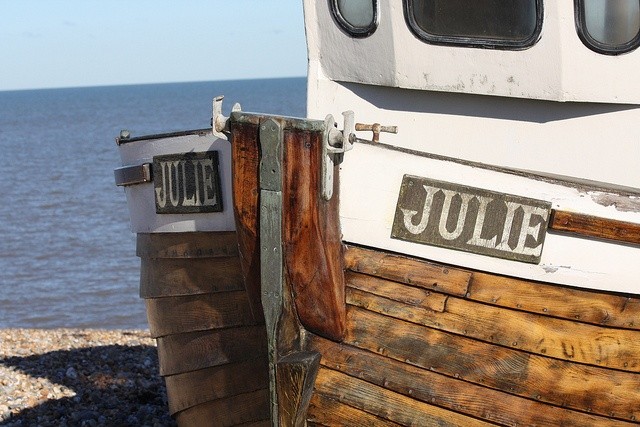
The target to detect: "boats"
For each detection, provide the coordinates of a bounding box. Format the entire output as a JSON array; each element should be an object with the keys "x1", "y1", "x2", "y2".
[{"x1": 113, "y1": 1, "x2": 638, "y2": 425}]
